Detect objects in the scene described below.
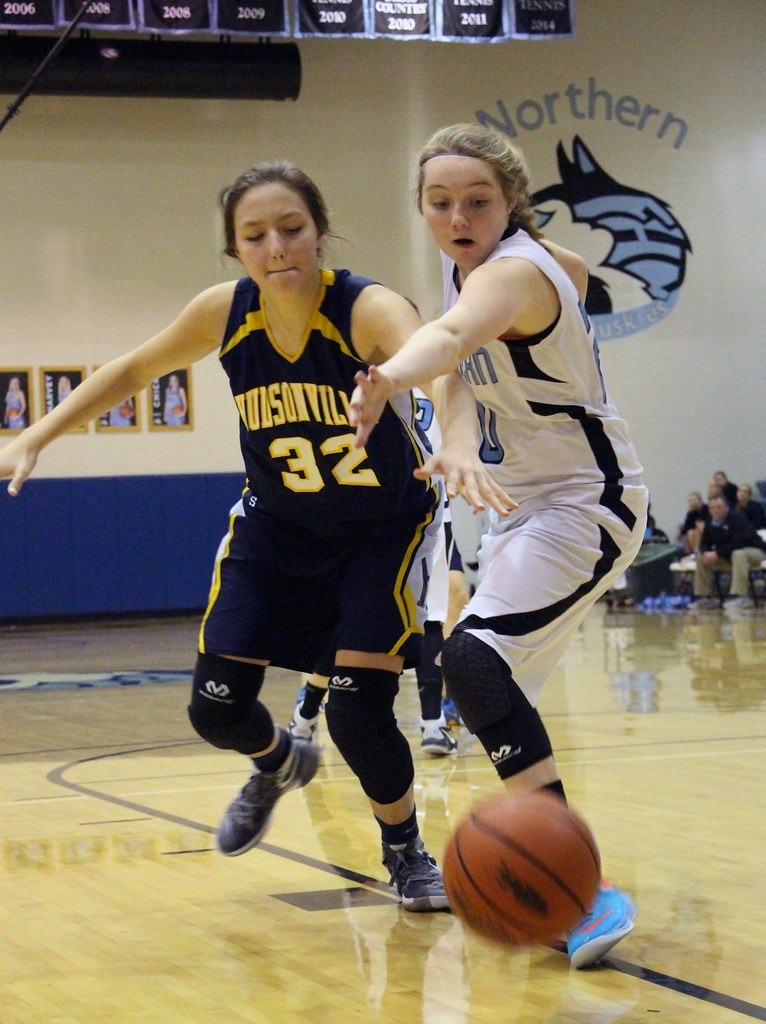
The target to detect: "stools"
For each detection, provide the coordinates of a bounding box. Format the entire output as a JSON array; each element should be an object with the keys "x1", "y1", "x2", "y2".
[{"x1": 713, "y1": 569, "x2": 766, "y2": 609}]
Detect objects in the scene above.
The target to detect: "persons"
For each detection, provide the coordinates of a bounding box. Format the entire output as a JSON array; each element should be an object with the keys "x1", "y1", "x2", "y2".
[
  {"x1": 57, "y1": 375, "x2": 73, "y2": 405},
  {"x1": 288, "y1": 293, "x2": 480, "y2": 754},
  {"x1": 3, "y1": 379, "x2": 27, "y2": 429},
  {"x1": 603, "y1": 471, "x2": 766, "y2": 612},
  {"x1": 108, "y1": 399, "x2": 134, "y2": 425},
  {"x1": 348, "y1": 120, "x2": 649, "y2": 971},
  {"x1": 0, "y1": 160, "x2": 518, "y2": 911},
  {"x1": 163, "y1": 374, "x2": 188, "y2": 426}
]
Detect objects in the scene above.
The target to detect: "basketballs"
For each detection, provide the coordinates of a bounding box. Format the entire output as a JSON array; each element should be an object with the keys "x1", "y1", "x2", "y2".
[{"x1": 443, "y1": 790, "x2": 604, "y2": 947}]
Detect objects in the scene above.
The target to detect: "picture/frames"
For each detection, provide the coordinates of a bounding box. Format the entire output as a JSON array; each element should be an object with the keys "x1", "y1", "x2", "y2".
[
  {"x1": 147, "y1": 363, "x2": 195, "y2": 434},
  {"x1": 92, "y1": 364, "x2": 143, "y2": 435},
  {"x1": 0, "y1": 364, "x2": 35, "y2": 437},
  {"x1": 38, "y1": 364, "x2": 89, "y2": 436}
]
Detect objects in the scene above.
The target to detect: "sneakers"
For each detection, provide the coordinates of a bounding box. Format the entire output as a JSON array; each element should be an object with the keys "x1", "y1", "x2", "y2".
[
  {"x1": 419, "y1": 711, "x2": 457, "y2": 755},
  {"x1": 287, "y1": 701, "x2": 321, "y2": 745},
  {"x1": 382, "y1": 834, "x2": 449, "y2": 912},
  {"x1": 296, "y1": 687, "x2": 325, "y2": 712},
  {"x1": 567, "y1": 875, "x2": 637, "y2": 969},
  {"x1": 442, "y1": 697, "x2": 465, "y2": 727},
  {"x1": 687, "y1": 597, "x2": 717, "y2": 610},
  {"x1": 217, "y1": 740, "x2": 319, "y2": 857},
  {"x1": 722, "y1": 597, "x2": 752, "y2": 611}
]
